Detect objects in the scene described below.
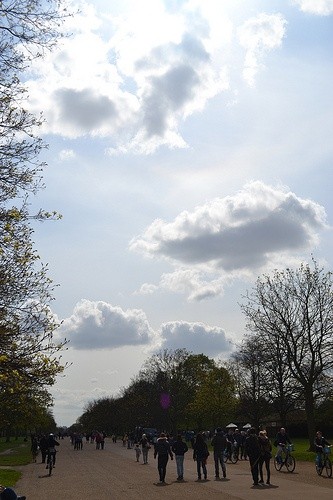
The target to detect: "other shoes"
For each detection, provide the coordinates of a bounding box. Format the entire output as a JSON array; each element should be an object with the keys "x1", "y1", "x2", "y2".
[
  {"x1": 319, "y1": 467, "x2": 322, "y2": 469},
  {"x1": 53, "y1": 465, "x2": 55, "y2": 468},
  {"x1": 266, "y1": 481, "x2": 270, "y2": 484},
  {"x1": 46, "y1": 465, "x2": 48, "y2": 469},
  {"x1": 276, "y1": 460, "x2": 279, "y2": 464}
]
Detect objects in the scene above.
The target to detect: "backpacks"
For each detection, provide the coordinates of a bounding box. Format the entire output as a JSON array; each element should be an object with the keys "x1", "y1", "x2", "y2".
[
  {"x1": 258, "y1": 436, "x2": 271, "y2": 452},
  {"x1": 197, "y1": 442, "x2": 208, "y2": 458},
  {"x1": 249, "y1": 436, "x2": 265, "y2": 457}
]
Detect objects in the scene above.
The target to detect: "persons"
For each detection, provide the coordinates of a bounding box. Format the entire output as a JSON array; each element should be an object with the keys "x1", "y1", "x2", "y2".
[
  {"x1": 154, "y1": 433, "x2": 173, "y2": 484},
  {"x1": 193, "y1": 434, "x2": 209, "y2": 482},
  {"x1": 225, "y1": 424, "x2": 333, "y2": 486},
  {"x1": 28, "y1": 424, "x2": 154, "y2": 476},
  {"x1": 211, "y1": 428, "x2": 228, "y2": 480},
  {"x1": 172, "y1": 435, "x2": 188, "y2": 480},
  {"x1": 0, "y1": 485, "x2": 26, "y2": 500}
]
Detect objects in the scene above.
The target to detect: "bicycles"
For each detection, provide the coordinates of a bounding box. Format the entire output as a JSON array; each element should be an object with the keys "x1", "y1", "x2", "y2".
[
  {"x1": 45, "y1": 451, "x2": 57, "y2": 476},
  {"x1": 274, "y1": 442, "x2": 295, "y2": 472},
  {"x1": 223, "y1": 442, "x2": 239, "y2": 463},
  {"x1": 315, "y1": 444, "x2": 333, "y2": 478}
]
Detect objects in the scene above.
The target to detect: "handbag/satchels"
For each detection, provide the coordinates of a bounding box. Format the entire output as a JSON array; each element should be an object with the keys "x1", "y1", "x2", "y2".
[
  {"x1": 273, "y1": 440, "x2": 278, "y2": 447},
  {"x1": 146, "y1": 442, "x2": 151, "y2": 449}
]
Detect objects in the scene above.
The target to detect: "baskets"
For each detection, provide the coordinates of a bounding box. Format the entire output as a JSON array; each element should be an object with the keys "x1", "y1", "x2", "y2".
[
  {"x1": 323, "y1": 448, "x2": 331, "y2": 454},
  {"x1": 284, "y1": 445, "x2": 295, "y2": 452}
]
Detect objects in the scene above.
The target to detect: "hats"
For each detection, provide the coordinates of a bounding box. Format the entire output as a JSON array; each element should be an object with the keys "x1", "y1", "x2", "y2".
[{"x1": 49, "y1": 433, "x2": 53, "y2": 437}]
